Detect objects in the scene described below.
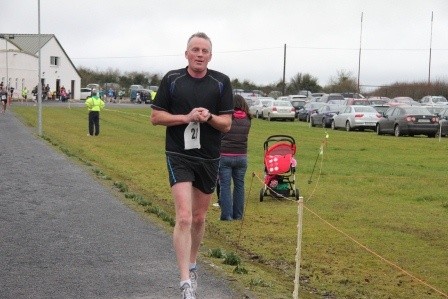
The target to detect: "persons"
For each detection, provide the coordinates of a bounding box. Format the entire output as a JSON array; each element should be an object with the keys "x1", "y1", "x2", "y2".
[
  {"x1": 135, "y1": 91, "x2": 142, "y2": 104},
  {"x1": 151, "y1": 33, "x2": 235, "y2": 299},
  {"x1": 23, "y1": 88, "x2": 27, "y2": 102},
  {"x1": 61, "y1": 87, "x2": 66, "y2": 102},
  {"x1": 34, "y1": 84, "x2": 49, "y2": 102},
  {"x1": 212, "y1": 172, "x2": 220, "y2": 206},
  {"x1": 85, "y1": 92, "x2": 104, "y2": 136},
  {"x1": 0, "y1": 82, "x2": 7, "y2": 112},
  {"x1": 109, "y1": 88, "x2": 125, "y2": 103},
  {"x1": 219, "y1": 95, "x2": 251, "y2": 221}
]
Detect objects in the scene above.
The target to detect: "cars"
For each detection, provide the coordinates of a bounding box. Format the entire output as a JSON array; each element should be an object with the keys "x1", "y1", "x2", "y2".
[
  {"x1": 233, "y1": 89, "x2": 448, "y2": 137},
  {"x1": 129, "y1": 85, "x2": 159, "y2": 103},
  {"x1": 80, "y1": 83, "x2": 100, "y2": 100}
]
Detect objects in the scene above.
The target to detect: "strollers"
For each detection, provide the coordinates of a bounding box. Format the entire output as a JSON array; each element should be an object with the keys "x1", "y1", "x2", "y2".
[{"x1": 260, "y1": 134, "x2": 299, "y2": 202}]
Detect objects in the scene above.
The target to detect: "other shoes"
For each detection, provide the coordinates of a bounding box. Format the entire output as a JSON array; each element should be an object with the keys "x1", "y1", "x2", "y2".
[{"x1": 212, "y1": 202, "x2": 220, "y2": 207}]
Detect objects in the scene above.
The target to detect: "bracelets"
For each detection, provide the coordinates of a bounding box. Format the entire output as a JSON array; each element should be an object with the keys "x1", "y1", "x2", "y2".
[{"x1": 207, "y1": 116, "x2": 212, "y2": 121}]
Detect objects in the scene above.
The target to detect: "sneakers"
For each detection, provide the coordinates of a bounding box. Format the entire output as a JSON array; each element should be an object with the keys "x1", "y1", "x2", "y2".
[{"x1": 179, "y1": 265, "x2": 200, "y2": 299}]
